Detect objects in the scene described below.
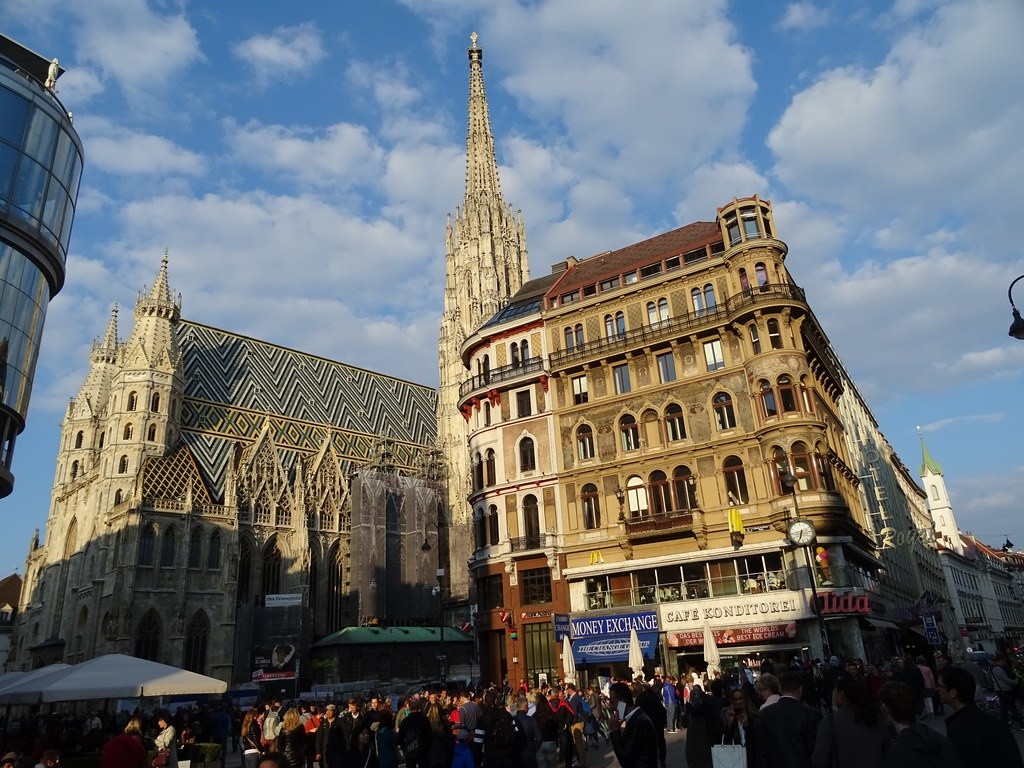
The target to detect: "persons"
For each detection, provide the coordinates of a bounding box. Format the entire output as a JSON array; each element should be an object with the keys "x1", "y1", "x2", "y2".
[
  {"x1": 960, "y1": 654, "x2": 987, "y2": 700},
  {"x1": 991, "y1": 657, "x2": 1023, "y2": 730},
  {"x1": 896, "y1": 654, "x2": 925, "y2": 720},
  {"x1": 474, "y1": 687, "x2": 527, "y2": 768},
  {"x1": 811, "y1": 673, "x2": 891, "y2": 768},
  {"x1": 724, "y1": 690, "x2": 756, "y2": 748},
  {"x1": 915, "y1": 656, "x2": 936, "y2": 720},
  {"x1": 0, "y1": 657, "x2": 872, "y2": 768},
  {"x1": 754, "y1": 673, "x2": 783, "y2": 712},
  {"x1": 607, "y1": 683, "x2": 658, "y2": 768},
  {"x1": 937, "y1": 668, "x2": 1024, "y2": 768},
  {"x1": 752, "y1": 672, "x2": 824, "y2": 768},
  {"x1": 878, "y1": 681, "x2": 964, "y2": 768}
]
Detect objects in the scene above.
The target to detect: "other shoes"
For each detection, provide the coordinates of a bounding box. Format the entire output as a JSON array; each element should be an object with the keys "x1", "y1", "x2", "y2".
[
  {"x1": 668, "y1": 730, "x2": 678, "y2": 733},
  {"x1": 606, "y1": 737, "x2": 611, "y2": 745},
  {"x1": 825, "y1": 709, "x2": 833, "y2": 714},
  {"x1": 919, "y1": 708, "x2": 944, "y2": 720},
  {"x1": 1009, "y1": 725, "x2": 1018, "y2": 732},
  {"x1": 675, "y1": 728, "x2": 681, "y2": 731}
]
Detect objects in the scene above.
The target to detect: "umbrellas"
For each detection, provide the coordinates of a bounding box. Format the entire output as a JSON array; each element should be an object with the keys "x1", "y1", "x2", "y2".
[
  {"x1": 628, "y1": 628, "x2": 645, "y2": 682},
  {"x1": 0, "y1": 672, "x2": 27, "y2": 688},
  {"x1": 0, "y1": 653, "x2": 227, "y2": 706},
  {"x1": 0, "y1": 664, "x2": 71, "y2": 692},
  {"x1": 702, "y1": 621, "x2": 722, "y2": 681},
  {"x1": 562, "y1": 636, "x2": 576, "y2": 685}
]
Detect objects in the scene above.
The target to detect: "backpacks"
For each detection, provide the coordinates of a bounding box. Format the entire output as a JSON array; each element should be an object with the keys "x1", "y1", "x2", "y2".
[
  {"x1": 491, "y1": 714, "x2": 513, "y2": 749},
  {"x1": 576, "y1": 695, "x2": 591, "y2": 714},
  {"x1": 263, "y1": 705, "x2": 284, "y2": 740}
]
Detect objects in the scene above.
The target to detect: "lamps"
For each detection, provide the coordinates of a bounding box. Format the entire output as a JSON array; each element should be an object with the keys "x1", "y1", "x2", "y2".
[
  {"x1": 686, "y1": 475, "x2": 697, "y2": 485},
  {"x1": 613, "y1": 485, "x2": 625, "y2": 503}
]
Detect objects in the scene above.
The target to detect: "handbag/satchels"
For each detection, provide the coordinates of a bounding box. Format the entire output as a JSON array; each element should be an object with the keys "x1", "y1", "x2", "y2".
[
  {"x1": 151, "y1": 742, "x2": 171, "y2": 768},
  {"x1": 1001, "y1": 693, "x2": 1017, "y2": 704},
  {"x1": 405, "y1": 728, "x2": 421, "y2": 758},
  {"x1": 711, "y1": 732, "x2": 747, "y2": 768}
]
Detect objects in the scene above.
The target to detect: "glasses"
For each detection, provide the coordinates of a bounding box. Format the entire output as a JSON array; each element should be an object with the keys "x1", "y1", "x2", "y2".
[{"x1": 935, "y1": 683, "x2": 949, "y2": 689}]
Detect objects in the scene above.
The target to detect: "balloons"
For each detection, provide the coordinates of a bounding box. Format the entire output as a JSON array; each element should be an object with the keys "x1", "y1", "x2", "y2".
[{"x1": 816, "y1": 547, "x2": 828, "y2": 562}]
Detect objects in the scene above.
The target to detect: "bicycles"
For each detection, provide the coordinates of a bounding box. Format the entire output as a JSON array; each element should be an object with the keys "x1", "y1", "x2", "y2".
[{"x1": 984, "y1": 694, "x2": 1014, "y2": 724}]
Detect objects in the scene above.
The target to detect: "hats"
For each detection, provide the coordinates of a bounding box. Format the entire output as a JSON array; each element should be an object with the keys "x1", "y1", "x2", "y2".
[
  {"x1": 102, "y1": 735, "x2": 147, "y2": 768},
  {"x1": 192, "y1": 705, "x2": 199, "y2": 710},
  {"x1": 830, "y1": 656, "x2": 838, "y2": 664},
  {"x1": 323, "y1": 704, "x2": 338, "y2": 712}
]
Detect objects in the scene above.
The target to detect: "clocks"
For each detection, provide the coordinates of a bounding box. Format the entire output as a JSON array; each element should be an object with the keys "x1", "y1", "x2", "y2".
[{"x1": 789, "y1": 520, "x2": 815, "y2": 546}]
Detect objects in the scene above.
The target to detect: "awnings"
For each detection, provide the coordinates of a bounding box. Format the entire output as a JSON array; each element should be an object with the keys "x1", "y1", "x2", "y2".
[{"x1": 866, "y1": 617, "x2": 899, "y2": 628}]
